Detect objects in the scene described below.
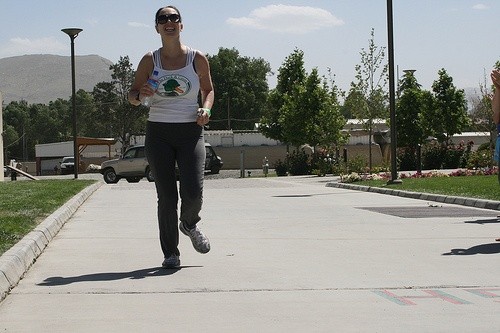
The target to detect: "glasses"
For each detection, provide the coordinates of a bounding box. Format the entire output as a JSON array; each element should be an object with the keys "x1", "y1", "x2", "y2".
[{"x1": 157, "y1": 15, "x2": 181, "y2": 24}]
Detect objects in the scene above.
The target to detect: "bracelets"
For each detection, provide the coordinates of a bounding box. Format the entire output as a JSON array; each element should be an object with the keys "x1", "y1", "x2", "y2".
[{"x1": 202, "y1": 108, "x2": 211, "y2": 117}]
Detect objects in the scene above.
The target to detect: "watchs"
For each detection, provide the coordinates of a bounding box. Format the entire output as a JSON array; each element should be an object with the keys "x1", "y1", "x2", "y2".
[{"x1": 135, "y1": 92, "x2": 141, "y2": 102}]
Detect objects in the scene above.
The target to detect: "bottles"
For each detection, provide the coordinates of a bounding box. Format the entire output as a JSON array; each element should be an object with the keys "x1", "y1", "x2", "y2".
[{"x1": 140, "y1": 70, "x2": 160, "y2": 108}]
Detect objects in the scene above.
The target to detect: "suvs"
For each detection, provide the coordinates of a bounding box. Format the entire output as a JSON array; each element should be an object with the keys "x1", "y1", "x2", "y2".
[{"x1": 100, "y1": 145, "x2": 180, "y2": 185}]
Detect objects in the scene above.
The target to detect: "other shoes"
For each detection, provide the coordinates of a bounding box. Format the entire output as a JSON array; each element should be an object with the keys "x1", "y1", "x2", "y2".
[
  {"x1": 162, "y1": 255, "x2": 180, "y2": 268},
  {"x1": 179, "y1": 222, "x2": 210, "y2": 253}
]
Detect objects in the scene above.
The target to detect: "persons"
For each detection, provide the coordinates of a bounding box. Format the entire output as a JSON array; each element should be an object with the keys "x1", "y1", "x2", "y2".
[
  {"x1": 317, "y1": 145, "x2": 329, "y2": 177},
  {"x1": 127, "y1": 6, "x2": 214, "y2": 269},
  {"x1": 490, "y1": 67, "x2": 500, "y2": 242},
  {"x1": 16, "y1": 160, "x2": 22, "y2": 177},
  {"x1": 262, "y1": 155, "x2": 269, "y2": 174},
  {"x1": 54, "y1": 161, "x2": 62, "y2": 173}
]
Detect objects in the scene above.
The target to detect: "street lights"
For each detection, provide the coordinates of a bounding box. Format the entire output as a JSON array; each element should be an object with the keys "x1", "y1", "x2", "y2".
[{"x1": 60, "y1": 27, "x2": 84, "y2": 178}]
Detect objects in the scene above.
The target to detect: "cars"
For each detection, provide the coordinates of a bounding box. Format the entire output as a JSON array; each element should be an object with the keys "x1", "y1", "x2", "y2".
[
  {"x1": 59, "y1": 156, "x2": 75, "y2": 174},
  {"x1": 205, "y1": 143, "x2": 224, "y2": 174}
]
